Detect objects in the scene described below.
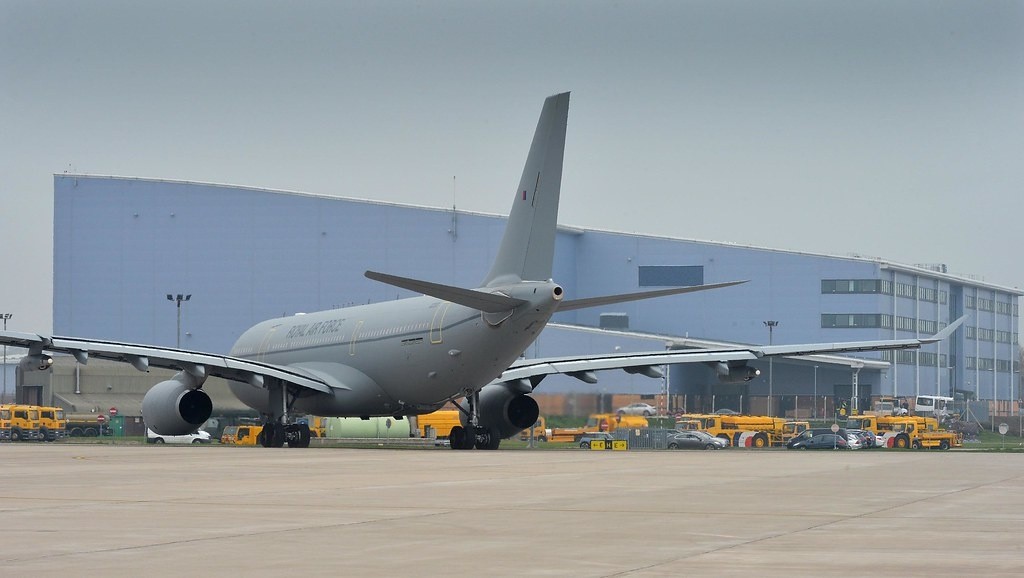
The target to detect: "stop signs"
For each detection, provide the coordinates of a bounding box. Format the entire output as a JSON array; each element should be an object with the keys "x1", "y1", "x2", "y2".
[
  {"x1": 97, "y1": 416, "x2": 105, "y2": 422},
  {"x1": 110, "y1": 408, "x2": 117, "y2": 415}
]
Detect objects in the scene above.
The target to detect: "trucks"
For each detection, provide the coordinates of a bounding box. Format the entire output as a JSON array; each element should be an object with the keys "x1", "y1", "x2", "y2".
[
  {"x1": 787, "y1": 427, "x2": 848, "y2": 450},
  {"x1": 845, "y1": 415, "x2": 963, "y2": 450},
  {"x1": 0, "y1": 96, "x2": 754, "y2": 450},
  {"x1": 0, "y1": 404, "x2": 264, "y2": 447},
  {"x1": 675, "y1": 413, "x2": 809, "y2": 447},
  {"x1": 520, "y1": 413, "x2": 649, "y2": 443}
]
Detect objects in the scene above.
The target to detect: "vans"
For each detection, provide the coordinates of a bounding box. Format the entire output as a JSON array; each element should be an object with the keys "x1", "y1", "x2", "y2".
[{"x1": 146, "y1": 428, "x2": 212, "y2": 445}]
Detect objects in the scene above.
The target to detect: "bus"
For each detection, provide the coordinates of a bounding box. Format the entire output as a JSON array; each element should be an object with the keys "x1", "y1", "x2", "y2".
[{"x1": 862, "y1": 394, "x2": 955, "y2": 417}]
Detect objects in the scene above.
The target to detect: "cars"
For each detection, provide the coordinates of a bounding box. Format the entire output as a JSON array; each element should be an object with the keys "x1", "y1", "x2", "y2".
[
  {"x1": 668, "y1": 432, "x2": 730, "y2": 450},
  {"x1": 793, "y1": 434, "x2": 846, "y2": 449},
  {"x1": 617, "y1": 402, "x2": 657, "y2": 416},
  {"x1": 716, "y1": 408, "x2": 742, "y2": 417},
  {"x1": 847, "y1": 429, "x2": 885, "y2": 450},
  {"x1": 580, "y1": 432, "x2": 615, "y2": 449}
]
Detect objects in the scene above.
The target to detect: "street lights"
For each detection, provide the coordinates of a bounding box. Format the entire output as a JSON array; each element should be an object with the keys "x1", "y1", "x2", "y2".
[
  {"x1": 762, "y1": 318, "x2": 779, "y2": 418},
  {"x1": 813, "y1": 365, "x2": 819, "y2": 419},
  {"x1": 0, "y1": 309, "x2": 15, "y2": 403},
  {"x1": 166, "y1": 293, "x2": 193, "y2": 349}
]
